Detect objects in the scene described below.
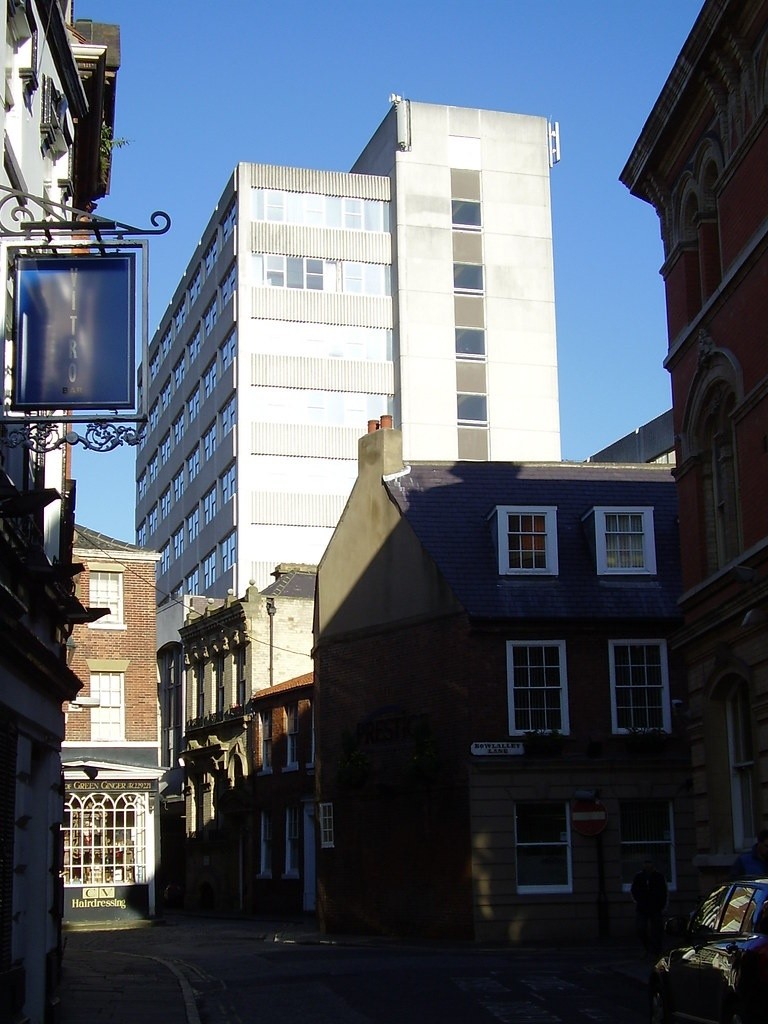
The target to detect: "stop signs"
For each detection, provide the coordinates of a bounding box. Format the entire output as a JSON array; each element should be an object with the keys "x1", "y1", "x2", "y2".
[{"x1": 572, "y1": 797, "x2": 609, "y2": 836}]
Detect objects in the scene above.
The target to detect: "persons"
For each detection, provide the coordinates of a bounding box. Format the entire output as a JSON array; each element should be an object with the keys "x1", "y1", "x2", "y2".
[
  {"x1": 632, "y1": 858, "x2": 667, "y2": 950},
  {"x1": 727, "y1": 830, "x2": 768, "y2": 879}
]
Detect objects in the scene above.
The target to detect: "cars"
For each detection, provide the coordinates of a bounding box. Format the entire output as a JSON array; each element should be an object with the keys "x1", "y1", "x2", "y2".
[
  {"x1": 159, "y1": 878, "x2": 187, "y2": 906},
  {"x1": 648, "y1": 877, "x2": 768, "y2": 1022}
]
